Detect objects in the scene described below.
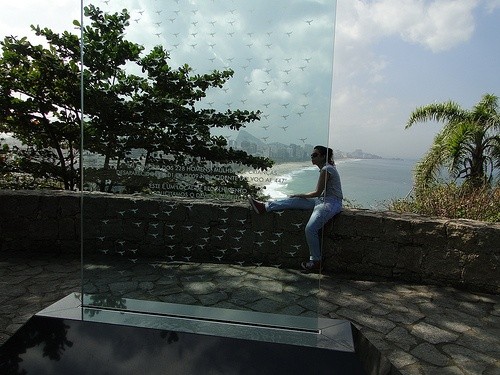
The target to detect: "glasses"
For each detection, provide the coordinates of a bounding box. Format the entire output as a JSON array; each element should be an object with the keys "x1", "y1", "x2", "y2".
[{"x1": 310, "y1": 153, "x2": 321, "y2": 157}]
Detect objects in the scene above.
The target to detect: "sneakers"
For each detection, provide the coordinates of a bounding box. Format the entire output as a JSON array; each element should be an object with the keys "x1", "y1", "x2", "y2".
[
  {"x1": 300, "y1": 261, "x2": 322, "y2": 273},
  {"x1": 247, "y1": 194, "x2": 266, "y2": 215}
]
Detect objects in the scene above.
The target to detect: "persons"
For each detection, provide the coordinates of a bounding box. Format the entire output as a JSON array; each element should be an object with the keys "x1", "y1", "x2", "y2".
[{"x1": 246, "y1": 144, "x2": 343, "y2": 271}]
